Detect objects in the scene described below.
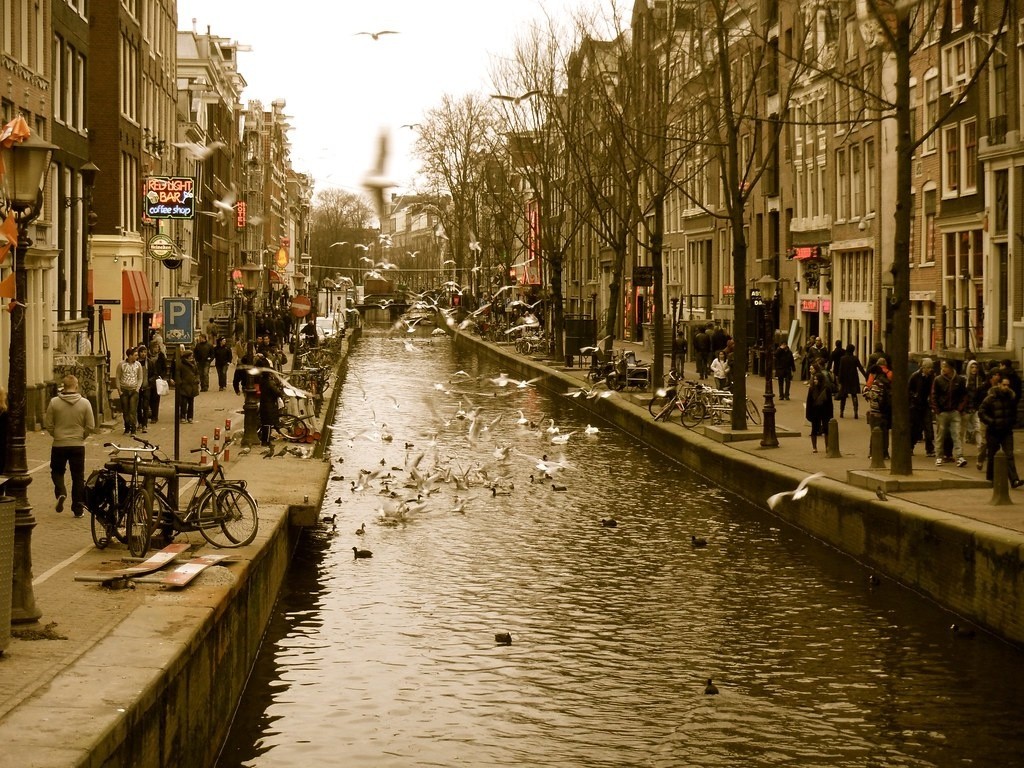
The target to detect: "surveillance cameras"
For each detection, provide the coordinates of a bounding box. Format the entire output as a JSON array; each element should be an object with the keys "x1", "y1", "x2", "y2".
[{"x1": 858, "y1": 223, "x2": 866, "y2": 231}]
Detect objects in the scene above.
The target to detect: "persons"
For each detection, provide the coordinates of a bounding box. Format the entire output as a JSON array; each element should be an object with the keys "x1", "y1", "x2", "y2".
[
  {"x1": 693, "y1": 323, "x2": 734, "y2": 390},
  {"x1": 773, "y1": 340, "x2": 796, "y2": 400},
  {"x1": 256, "y1": 307, "x2": 296, "y2": 354},
  {"x1": 232, "y1": 336, "x2": 288, "y2": 439},
  {"x1": 233, "y1": 314, "x2": 244, "y2": 343},
  {"x1": 804, "y1": 337, "x2": 892, "y2": 459},
  {"x1": 194, "y1": 318, "x2": 233, "y2": 391},
  {"x1": 676, "y1": 331, "x2": 688, "y2": 379},
  {"x1": 269, "y1": 285, "x2": 293, "y2": 308},
  {"x1": 169, "y1": 344, "x2": 200, "y2": 422},
  {"x1": 909, "y1": 357, "x2": 1024, "y2": 487},
  {"x1": 300, "y1": 322, "x2": 319, "y2": 352},
  {"x1": 420, "y1": 287, "x2": 426, "y2": 300},
  {"x1": 46, "y1": 375, "x2": 95, "y2": 517},
  {"x1": 116, "y1": 335, "x2": 167, "y2": 435},
  {"x1": 481, "y1": 294, "x2": 545, "y2": 330}
]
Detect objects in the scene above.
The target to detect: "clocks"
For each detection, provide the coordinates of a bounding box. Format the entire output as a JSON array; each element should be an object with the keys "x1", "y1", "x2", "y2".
[{"x1": 0, "y1": 187, "x2": 43, "y2": 223}]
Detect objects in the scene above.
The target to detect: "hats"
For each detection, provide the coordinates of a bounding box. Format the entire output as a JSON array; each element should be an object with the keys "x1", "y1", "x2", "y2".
[
  {"x1": 965, "y1": 360, "x2": 979, "y2": 390},
  {"x1": 126, "y1": 346, "x2": 138, "y2": 356},
  {"x1": 942, "y1": 360, "x2": 954, "y2": 369},
  {"x1": 922, "y1": 358, "x2": 933, "y2": 367},
  {"x1": 182, "y1": 350, "x2": 192, "y2": 357}
]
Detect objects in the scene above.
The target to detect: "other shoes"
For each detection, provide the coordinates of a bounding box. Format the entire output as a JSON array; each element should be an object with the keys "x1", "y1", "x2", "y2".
[
  {"x1": 840, "y1": 414, "x2": 843, "y2": 418},
  {"x1": 779, "y1": 396, "x2": 783, "y2": 400},
  {"x1": 910, "y1": 447, "x2": 982, "y2": 468},
  {"x1": 124, "y1": 419, "x2": 158, "y2": 437},
  {"x1": 1011, "y1": 479, "x2": 1024, "y2": 488},
  {"x1": 785, "y1": 396, "x2": 789, "y2": 400},
  {"x1": 188, "y1": 418, "x2": 193, "y2": 423},
  {"x1": 200, "y1": 386, "x2": 208, "y2": 392},
  {"x1": 56, "y1": 495, "x2": 66, "y2": 513},
  {"x1": 219, "y1": 386, "x2": 226, "y2": 391},
  {"x1": 180, "y1": 418, "x2": 186, "y2": 424},
  {"x1": 75, "y1": 512, "x2": 84, "y2": 517},
  {"x1": 855, "y1": 415, "x2": 858, "y2": 418}
]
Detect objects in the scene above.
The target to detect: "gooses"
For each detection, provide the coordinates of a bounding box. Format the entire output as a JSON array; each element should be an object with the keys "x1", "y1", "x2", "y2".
[
  {"x1": 579, "y1": 335, "x2": 611, "y2": 354},
  {"x1": 584, "y1": 424, "x2": 601, "y2": 436}
]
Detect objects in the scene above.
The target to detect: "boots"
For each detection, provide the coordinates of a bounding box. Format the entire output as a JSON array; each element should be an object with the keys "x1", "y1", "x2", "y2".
[
  {"x1": 811, "y1": 436, "x2": 818, "y2": 453},
  {"x1": 825, "y1": 435, "x2": 828, "y2": 453}
]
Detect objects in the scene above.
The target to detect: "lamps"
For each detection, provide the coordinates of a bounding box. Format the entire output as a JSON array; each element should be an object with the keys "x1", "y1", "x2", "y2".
[{"x1": 61, "y1": 157, "x2": 102, "y2": 212}]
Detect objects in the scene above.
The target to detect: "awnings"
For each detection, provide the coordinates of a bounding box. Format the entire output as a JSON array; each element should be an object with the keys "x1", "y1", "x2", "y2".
[
  {"x1": 122, "y1": 269, "x2": 152, "y2": 313},
  {"x1": 270, "y1": 270, "x2": 284, "y2": 285}
]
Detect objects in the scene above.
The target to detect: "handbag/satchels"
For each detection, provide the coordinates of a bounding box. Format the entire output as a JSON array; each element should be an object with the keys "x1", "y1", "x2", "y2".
[
  {"x1": 80, "y1": 469, "x2": 128, "y2": 512},
  {"x1": 155, "y1": 376, "x2": 169, "y2": 395},
  {"x1": 834, "y1": 386, "x2": 848, "y2": 400}
]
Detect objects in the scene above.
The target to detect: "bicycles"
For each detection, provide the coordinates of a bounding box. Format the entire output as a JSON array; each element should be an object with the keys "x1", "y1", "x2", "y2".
[
  {"x1": 263, "y1": 337, "x2": 341, "y2": 440},
  {"x1": 649, "y1": 369, "x2": 762, "y2": 428},
  {"x1": 472, "y1": 316, "x2": 552, "y2": 356},
  {"x1": 587, "y1": 355, "x2": 651, "y2": 391},
  {"x1": 78, "y1": 434, "x2": 259, "y2": 558}
]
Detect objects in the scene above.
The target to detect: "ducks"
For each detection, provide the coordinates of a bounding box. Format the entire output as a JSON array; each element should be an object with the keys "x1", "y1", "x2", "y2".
[
  {"x1": 329, "y1": 423, "x2": 515, "y2": 504},
  {"x1": 356, "y1": 523, "x2": 365, "y2": 536},
  {"x1": 691, "y1": 535, "x2": 708, "y2": 547},
  {"x1": 352, "y1": 547, "x2": 373, "y2": 559},
  {"x1": 529, "y1": 455, "x2": 568, "y2": 492},
  {"x1": 323, "y1": 514, "x2": 337, "y2": 523},
  {"x1": 327, "y1": 525, "x2": 338, "y2": 537},
  {"x1": 494, "y1": 632, "x2": 512, "y2": 646},
  {"x1": 335, "y1": 497, "x2": 342, "y2": 503},
  {"x1": 705, "y1": 679, "x2": 719, "y2": 694},
  {"x1": 601, "y1": 519, "x2": 617, "y2": 528}
]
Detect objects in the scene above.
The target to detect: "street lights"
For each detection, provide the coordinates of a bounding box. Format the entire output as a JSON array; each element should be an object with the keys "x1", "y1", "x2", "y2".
[
  {"x1": 238, "y1": 258, "x2": 265, "y2": 446},
  {"x1": 0, "y1": 112, "x2": 60, "y2": 633},
  {"x1": 588, "y1": 280, "x2": 601, "y2": 367},
  {"x1": 323, "y1": 277, "x2": 334, "y2": 318},
  {"x1": 666, "y1": 277, "x2": 685, "y2": 386},
  {"x1": 755, "y1": 274, "x2": 780, "y2": 446},
  {"x1": 291, "y1": 271, "x2": 306, "y2": 370}
]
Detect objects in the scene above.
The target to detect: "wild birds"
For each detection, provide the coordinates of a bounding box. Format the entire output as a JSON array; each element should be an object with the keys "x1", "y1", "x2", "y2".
[
  {"x1": 243, "y1": 226, "x2": 675, "y2": 531},
  {"x1": 489, "y1": 89, "x2": 543, "y2": 104},
  {"x1": 402, "y1": 123, "x2": 420, "y2": 130},
  {"x1": 354, "y1": 29, "x2": 401, "y2": 40},
  {"x1": 767, "y1": 471, "x2": 827, "y2": 511}
]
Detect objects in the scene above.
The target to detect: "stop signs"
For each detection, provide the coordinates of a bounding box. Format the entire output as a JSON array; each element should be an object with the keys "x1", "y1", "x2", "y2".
[
  {"x1": 291, "y1": 296, "x2": 311, "y2": 317},
  {"x1": 511, "y1": 275, "x2": 517, "y2": 285}
]
